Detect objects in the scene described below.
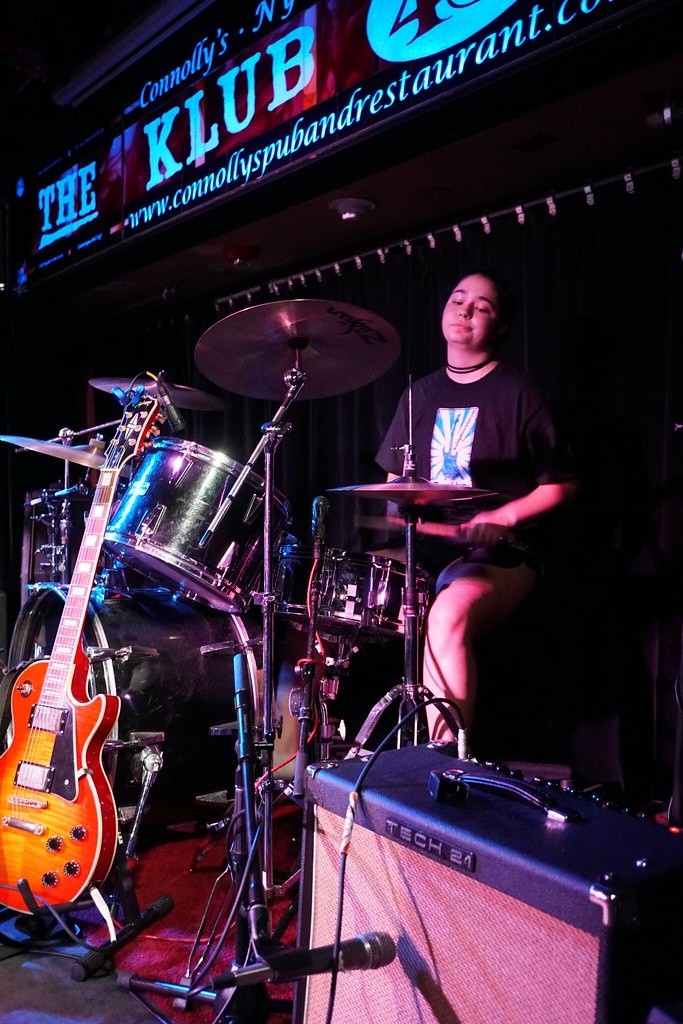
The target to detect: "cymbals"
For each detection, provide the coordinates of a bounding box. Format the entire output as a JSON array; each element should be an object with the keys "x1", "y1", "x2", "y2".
[
  {"x1": 324, "y1": 481, "x2": 502, "y2": 504},
  {"x1": 0, "y1": 430, "x2": 136, "y2": 477},
  {"x1": 193, "y1": 298, "x2": 399, "y2": 401},
  {"x1": 89, "y1": 377, "x2": 230, "y2": 417}
]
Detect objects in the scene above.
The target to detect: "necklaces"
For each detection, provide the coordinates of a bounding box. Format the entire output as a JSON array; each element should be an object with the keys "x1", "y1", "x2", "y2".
[{"x1": 447, "y1": 355, "x2": 494, "y2": 373}]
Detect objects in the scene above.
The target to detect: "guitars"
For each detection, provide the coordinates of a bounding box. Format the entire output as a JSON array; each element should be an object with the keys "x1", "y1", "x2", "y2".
[{"x1": 0, "y1": 393, "x2": 161, "y2": 918}]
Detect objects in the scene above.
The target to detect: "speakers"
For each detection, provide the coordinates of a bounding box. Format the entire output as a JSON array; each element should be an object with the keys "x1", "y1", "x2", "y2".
[{"x1": 304, "y1": 744, "x2": 683, "y2": 1024}]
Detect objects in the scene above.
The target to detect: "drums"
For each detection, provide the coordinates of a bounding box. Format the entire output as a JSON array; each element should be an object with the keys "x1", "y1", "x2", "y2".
[
  {"x1": 10, "y1": 493, "x2": 258, "y2": 806},
  {"x1": 101, "y1": 440, "x2": 287, "y2": 613},
  {"x1": 275, "y1": 546, "x2": 432, "y2": 647}
]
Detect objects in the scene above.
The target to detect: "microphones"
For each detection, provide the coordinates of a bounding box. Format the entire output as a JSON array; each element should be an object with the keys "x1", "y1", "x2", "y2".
[
  {"x1": 210, "y1": 932, "x2": 396, "y2": 991},
  {"x1": 309, "y1": 496, "x2": 330, "y2": 538},
  {"x1": 156, "y1": 381, "x2": 188, "y2": 433}
]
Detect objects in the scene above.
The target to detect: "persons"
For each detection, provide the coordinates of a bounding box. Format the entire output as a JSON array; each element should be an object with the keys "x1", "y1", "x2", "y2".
[{"x1": 270, "y1": 271, "x2": 570, "y2": 779}]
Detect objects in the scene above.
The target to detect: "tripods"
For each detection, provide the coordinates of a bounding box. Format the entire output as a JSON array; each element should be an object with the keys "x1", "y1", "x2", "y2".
[
  {"x1": 342, "y1": 503, "x2": 468, "y2": 760},
  {"x1": 117, "y1": 649, "x2": 295, "y2": 1024}
]
